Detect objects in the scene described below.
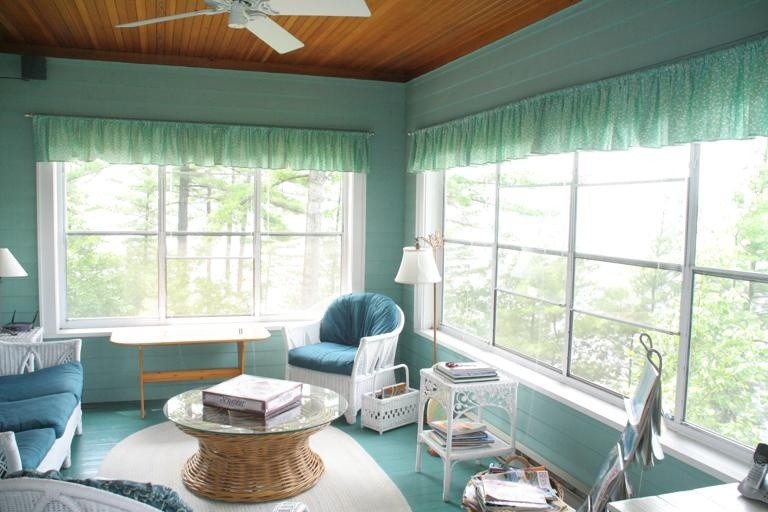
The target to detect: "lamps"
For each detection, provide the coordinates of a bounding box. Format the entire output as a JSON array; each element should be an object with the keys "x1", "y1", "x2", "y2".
[
  {"x1": 0, "y1": 246, "x2": 28, "y2": 337},
  {"x1": 393, "y1": 231, "x2": 444, "y2": 365},
  {"x1": 226, "y1": 0, "x2": 249, "y2": 30}
]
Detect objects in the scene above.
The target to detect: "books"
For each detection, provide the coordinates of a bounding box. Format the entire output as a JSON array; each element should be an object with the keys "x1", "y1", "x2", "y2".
[
  {"x1": 579, "y1": 360, "x2": 667, "y2": 512},
  {"x1": 381, "y1": 382, "x2": 406, "y2": 398},
  {"x1": 460, "y1": 462, "x2": 577, "y2": 512},
  {"x1": 434, "y1": 360, "x2": 501, "y2": 383},
  {"x1": 428, "y1": 418, "x2": 496, "y2": 449}
]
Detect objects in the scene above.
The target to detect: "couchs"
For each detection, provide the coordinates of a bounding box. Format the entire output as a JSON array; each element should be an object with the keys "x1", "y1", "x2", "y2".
[{"x1": 0, "y1": 339, "x2": 85, "y2": 477}]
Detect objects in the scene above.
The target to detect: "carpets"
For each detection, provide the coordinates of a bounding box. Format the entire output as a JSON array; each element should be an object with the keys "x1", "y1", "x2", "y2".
[{"x1": 99, "y1": 420, "x2": 410, "y2": 512}]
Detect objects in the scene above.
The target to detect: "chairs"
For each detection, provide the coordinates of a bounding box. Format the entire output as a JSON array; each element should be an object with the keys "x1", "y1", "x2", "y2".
[{"x1": 281, "y1": 291, "x2": 406, "y2": 425}]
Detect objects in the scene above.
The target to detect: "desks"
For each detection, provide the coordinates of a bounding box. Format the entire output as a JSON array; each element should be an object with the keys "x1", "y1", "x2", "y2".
[
  {"x1": 0, "y1": 325, "x2": 43, "y2": 341},
  {"x1": 606, "y1": 481, "x2": 767, "y2": 512},
  {"x1": 417, "y1": 368, "x2": 519, "y2": 503},
  {"x1": 109, "y1": 321, "x2": 271, "y2": 419}
]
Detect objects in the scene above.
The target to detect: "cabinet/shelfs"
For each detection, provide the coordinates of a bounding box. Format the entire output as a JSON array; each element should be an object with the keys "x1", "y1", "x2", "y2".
[{"x1": 574, "y1": 332, "x2": 664, "y2": 512}]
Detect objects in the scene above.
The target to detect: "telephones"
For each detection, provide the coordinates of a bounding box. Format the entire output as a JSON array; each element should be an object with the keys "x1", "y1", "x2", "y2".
[{"x1": 737, "y1": 443, "x2": 768, "y2": 504}]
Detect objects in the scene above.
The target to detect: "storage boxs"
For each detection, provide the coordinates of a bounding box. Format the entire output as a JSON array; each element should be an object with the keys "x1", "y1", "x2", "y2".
[{"x1": 361, "y1": 363, "x2": 419, "y2": 435}]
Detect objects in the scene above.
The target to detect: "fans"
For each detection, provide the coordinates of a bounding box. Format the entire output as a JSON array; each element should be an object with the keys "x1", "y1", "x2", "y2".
[{"x1": 111, "y1": 0, "x2": 372, "y2": 56}]
